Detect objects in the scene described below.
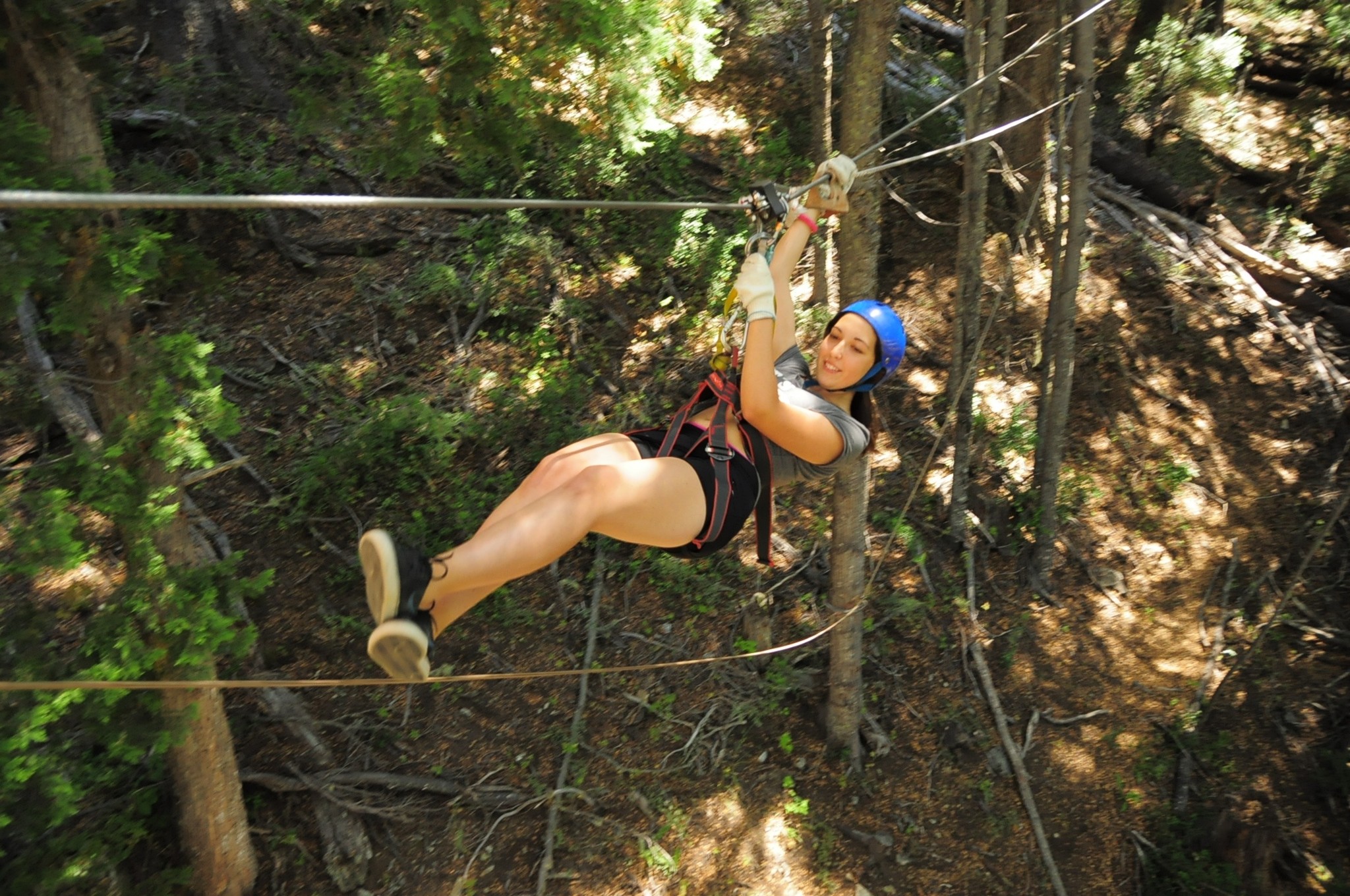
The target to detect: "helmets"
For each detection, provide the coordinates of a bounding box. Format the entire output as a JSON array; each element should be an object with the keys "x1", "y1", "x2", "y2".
[{"x1": 841, "y1": 298, "x2": 906, "y2": 392}]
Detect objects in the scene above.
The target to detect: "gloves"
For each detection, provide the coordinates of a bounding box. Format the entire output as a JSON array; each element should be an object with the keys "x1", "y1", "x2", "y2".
[
  {"x1": 734, "y1": 252, "x2": 775, "y2": 323},
  {"x1": 812, "y1": 154, "x2": 858, "y2": 218}
]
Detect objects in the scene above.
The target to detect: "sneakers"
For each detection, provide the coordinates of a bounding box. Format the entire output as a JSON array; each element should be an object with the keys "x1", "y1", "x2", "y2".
[
  {"x1": 358, "y1": 527, "x2": 454, "y2": 628},
  {"x1": 367, "y1": 599, "x2": 436, "y2": 684}
]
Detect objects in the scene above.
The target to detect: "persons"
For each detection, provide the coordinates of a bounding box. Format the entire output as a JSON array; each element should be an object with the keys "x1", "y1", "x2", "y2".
[{"x1": 359, "y1": 155, "x2": 905, "y2": 683}]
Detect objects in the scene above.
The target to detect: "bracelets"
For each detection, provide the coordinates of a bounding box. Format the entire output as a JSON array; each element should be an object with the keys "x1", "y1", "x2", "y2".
[{"x1": 793, "y1": 214, "x2": 818, "y2": 233}]
